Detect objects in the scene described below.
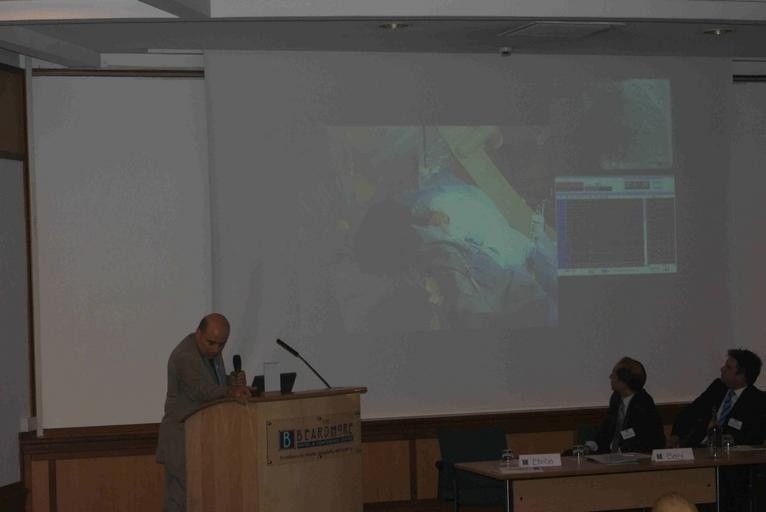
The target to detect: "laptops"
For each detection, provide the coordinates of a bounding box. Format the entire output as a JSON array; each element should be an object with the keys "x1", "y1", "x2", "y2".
[{"x1": 251, "y1": 372, "x2": 297, "y2": 398}]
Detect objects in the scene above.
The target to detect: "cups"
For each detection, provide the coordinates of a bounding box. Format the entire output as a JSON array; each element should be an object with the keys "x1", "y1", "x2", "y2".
[
  {"x1": 722, "y1": 435, "x2": 735, "y2": 460},
  {"x1": 502, "y1": 450, "x2": 514, "y2": 469},
  {"x1": 572, "y1": 445, "x2": 584, "y2": 466}
]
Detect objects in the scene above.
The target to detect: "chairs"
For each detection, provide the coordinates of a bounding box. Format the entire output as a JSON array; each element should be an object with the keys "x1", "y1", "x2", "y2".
[{"x1": 432, "y1": 425, "x2": 611, "y2": 512}]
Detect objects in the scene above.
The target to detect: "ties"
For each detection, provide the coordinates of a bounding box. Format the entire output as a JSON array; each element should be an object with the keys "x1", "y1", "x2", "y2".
[
  {"x1": 610, "y1": 401, "x2": 625, "y2": 452},
  {"x1": 719, "y1": 390, "x2": 732, "y2": 428}
]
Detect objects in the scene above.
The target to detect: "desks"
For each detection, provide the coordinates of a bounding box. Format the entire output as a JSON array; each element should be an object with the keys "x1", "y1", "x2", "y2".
[{"x1": 454, "y1": 444, "x2": 765, "y2": 511}]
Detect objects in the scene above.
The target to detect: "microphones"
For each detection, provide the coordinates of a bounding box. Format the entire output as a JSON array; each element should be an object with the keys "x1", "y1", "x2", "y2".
[
  {"x1": 276, "y1": 338, "x2": 332, "y2": 388},
  {"x1": 232, "y1": 354, "x2": 242, "y2": 377}
]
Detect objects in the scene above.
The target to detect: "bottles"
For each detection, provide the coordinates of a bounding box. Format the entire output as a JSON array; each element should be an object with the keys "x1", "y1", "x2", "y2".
[{"x1": 706, "y1": 421, "x2": 723, "y2": 458}]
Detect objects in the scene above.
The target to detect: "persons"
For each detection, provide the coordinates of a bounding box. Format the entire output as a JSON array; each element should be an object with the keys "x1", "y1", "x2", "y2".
[
  {"x1": 583, "y1": 357, "x2": 664, "y2": 454},
  {"x1": 669, "y1": 349, "x2": 766, "y2": 512},
  {"x1": 155, "y1": 314, "x2": 258, "y2": 512},
  {"x1": 654, "y1": 493, "x2": 697, "y2": 512}
]
